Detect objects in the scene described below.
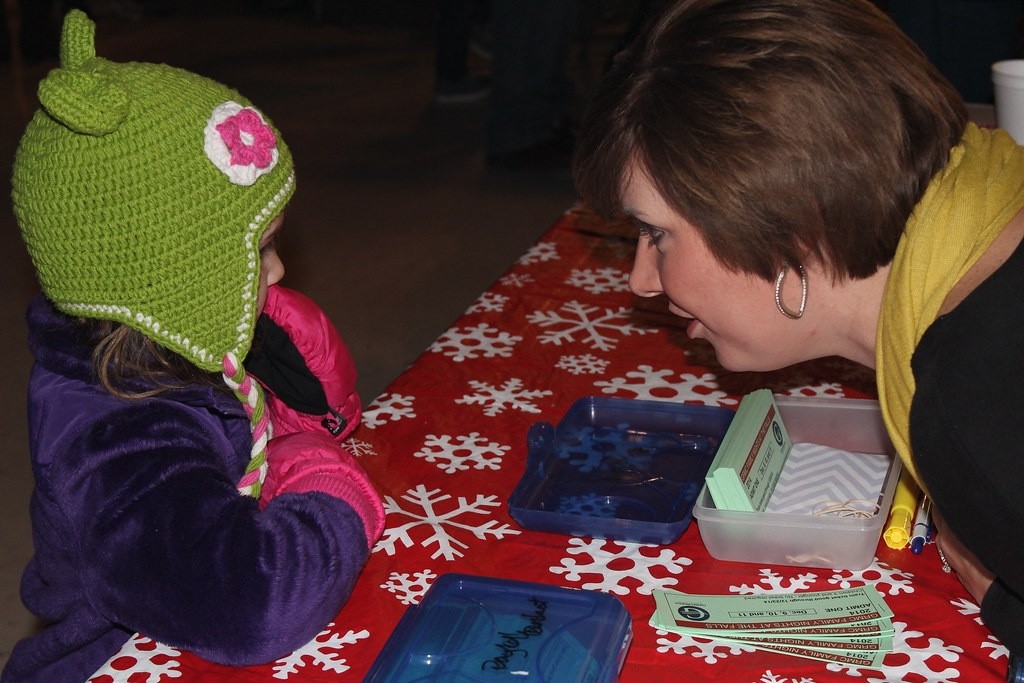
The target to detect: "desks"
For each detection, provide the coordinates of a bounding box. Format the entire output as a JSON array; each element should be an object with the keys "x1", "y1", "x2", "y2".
[{"x1": 82, "y1": 181, "x2": 1012, "y2": 683}]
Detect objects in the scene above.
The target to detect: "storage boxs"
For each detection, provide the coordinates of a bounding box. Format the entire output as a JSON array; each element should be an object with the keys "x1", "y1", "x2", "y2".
[{"x1": 691, "y1": 394, "x2": 904, "y2": 572}]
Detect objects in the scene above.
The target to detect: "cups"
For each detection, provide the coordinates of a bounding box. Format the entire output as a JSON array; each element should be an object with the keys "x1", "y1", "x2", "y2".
[{"x1": 991, "y1": 59, "x2": 1024, "y2": 146}]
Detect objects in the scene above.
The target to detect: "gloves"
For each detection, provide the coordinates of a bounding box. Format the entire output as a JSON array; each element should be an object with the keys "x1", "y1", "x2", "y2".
[
  {"x1": 242, "y1": 283, "x2": 362, "y2": 442},
  {"x1": 259, "y1": 428, "x2": 387, "y2": 557}
]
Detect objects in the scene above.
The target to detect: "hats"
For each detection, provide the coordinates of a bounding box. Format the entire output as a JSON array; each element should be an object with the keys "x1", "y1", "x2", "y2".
[{"x1": 12, "y1": 9, "x2": 296, "y2": 500}]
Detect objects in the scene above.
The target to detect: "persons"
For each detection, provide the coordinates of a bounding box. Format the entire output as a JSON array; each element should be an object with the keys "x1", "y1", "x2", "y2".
[
  {"x1": 574, "y1": 1, "x2": 1024, "y2": 659},
  {"x1": 889, "y1": 0, "x2": 1016, "y2": 104},
  {"x1": 0, "y1": 10, "x2": 384, "y2": 683},
  {"x1": 434, "y1": 0, "x2": 589, "y2": 166}
]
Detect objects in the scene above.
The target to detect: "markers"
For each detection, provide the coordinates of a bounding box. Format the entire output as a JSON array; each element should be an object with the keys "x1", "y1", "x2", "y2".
[{"x1": 883, "y1": 463, "x2": 920, "y2": 550}]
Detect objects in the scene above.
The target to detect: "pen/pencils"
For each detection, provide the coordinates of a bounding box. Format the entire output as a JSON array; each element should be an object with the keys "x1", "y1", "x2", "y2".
[{"x1": 909, "y1": 494, "x2": 932, "y2": 555}]
